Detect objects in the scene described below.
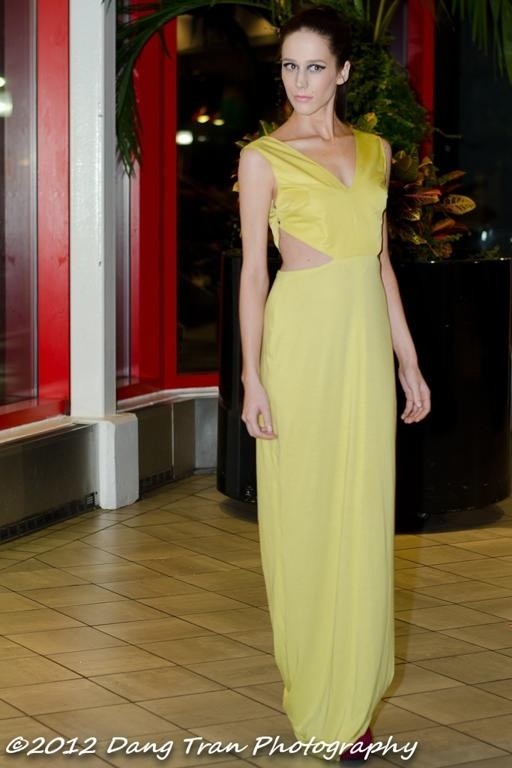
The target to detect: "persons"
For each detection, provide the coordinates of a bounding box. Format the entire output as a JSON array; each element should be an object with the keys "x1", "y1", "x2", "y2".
[{"x1": 238, "y1": 4, "x2": 434, "y2": 764}]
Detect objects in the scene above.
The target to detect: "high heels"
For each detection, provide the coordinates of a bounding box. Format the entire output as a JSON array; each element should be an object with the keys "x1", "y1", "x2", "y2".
[
  {"x1": 355, "y1": 727, "x2": 373, "y2": 748},
  {"x1": 340, "y1": 747, "x2": 364, "y2": 760}
]
